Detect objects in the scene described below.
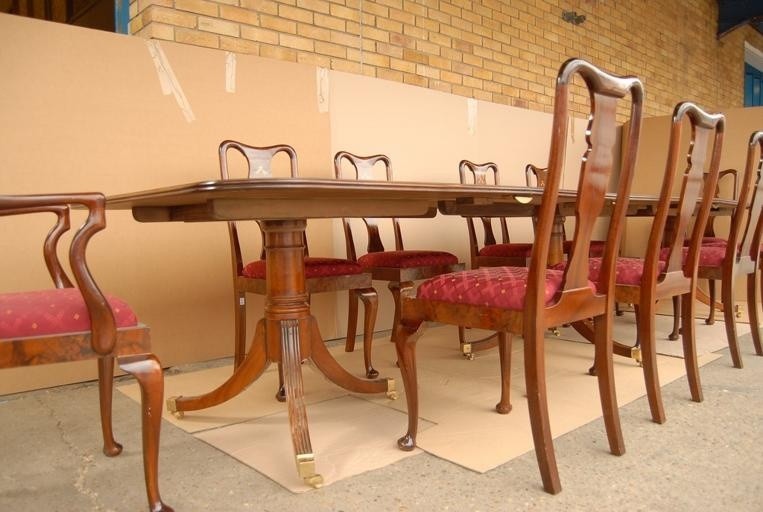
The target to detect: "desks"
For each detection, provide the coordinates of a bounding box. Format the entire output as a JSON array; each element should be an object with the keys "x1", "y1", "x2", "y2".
[{"x1": 68, "y1": 181, "x2": 746, "y2": 414}]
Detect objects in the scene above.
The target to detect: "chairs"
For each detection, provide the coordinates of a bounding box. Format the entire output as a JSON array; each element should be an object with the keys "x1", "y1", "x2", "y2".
[
  {"x1": 1, "y1": 188, "x2": 176, "y2": 512},
  {"x1": 217, "y1": 135, "x2": 386, "y2": 379},
  {"x1": 666, "y1": 127, "x2": 760, "y2": 365},
  {"x1": 456, "y1": 155, "x2": 529, "y2": 358},
  {"x1": 705, "y1": 168, "x2": 739, "y2": 202},
  {"x1": 555, "y1": 97, "x2": 726, "y2": 426},
  {"x1": 524, "y1": 159, "x2": 556, "y2": 188},
  {"x1": 391, "y1": 57, "x2": 646, "y2": 495},
  {"x1": 332, "y1": 148, "x2": 469, "y2": 375}
]
